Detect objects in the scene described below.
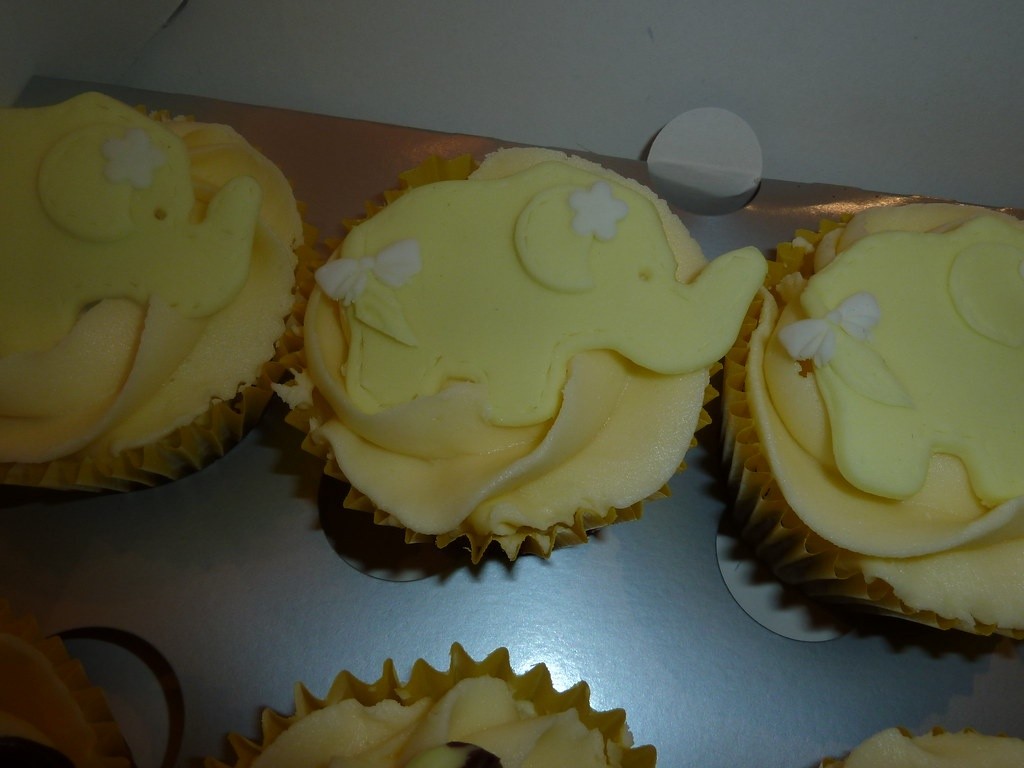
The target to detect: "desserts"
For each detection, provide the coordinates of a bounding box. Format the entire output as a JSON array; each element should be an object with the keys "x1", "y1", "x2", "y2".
[
  {"x1": 213, "y1": 644, "x2": 659, "y2": 768},
  {"x1": 0, "y1": 90, "x2": 1020, "y2": 639}
]
